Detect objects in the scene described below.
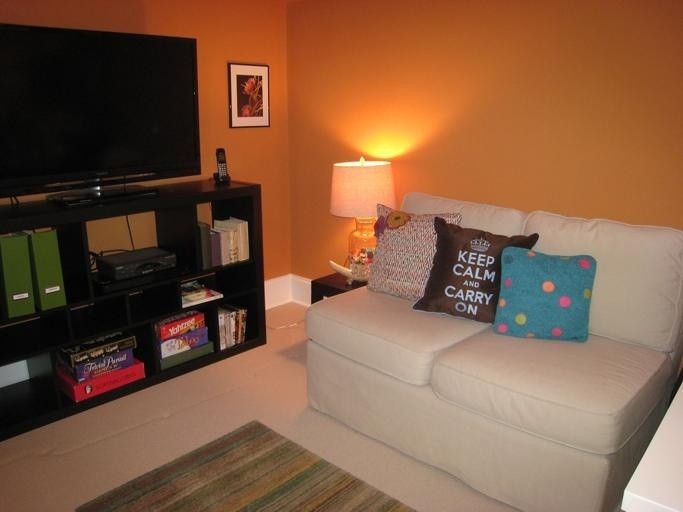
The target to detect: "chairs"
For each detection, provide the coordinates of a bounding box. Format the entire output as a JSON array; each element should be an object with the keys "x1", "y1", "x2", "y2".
[{"x1": 304, "y1": 193, "x2": 682, "y2": 510}]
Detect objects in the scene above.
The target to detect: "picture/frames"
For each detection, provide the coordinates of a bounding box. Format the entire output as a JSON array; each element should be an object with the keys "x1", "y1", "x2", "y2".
[{"x1": 225, "y1": 61, "x2": 272, "y2": 130}]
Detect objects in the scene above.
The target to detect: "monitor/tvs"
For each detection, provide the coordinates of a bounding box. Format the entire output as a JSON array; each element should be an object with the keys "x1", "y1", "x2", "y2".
[{"x1": 0, "y1": 24, "x2": 201, "y2": 207}]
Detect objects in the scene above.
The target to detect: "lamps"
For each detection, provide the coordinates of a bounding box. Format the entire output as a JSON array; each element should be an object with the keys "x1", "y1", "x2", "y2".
[{"x1": 328, "y1": 157, "x2": 397, "y2": 277}]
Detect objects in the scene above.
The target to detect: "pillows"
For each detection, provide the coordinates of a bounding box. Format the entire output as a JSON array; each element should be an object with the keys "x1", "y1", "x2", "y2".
[
  {"x1": 494, "y1": 245, "x2": 598, "y2": 344},
  {"x1": 365, "y1": 202, "x2": 462, "y2": 300},
  {"x1": 413, "y1": 215, "x2": 539, "y2": 324}
]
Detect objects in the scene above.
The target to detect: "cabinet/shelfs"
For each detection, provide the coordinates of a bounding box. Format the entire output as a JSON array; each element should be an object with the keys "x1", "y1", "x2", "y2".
[
  {"x1": 310, "y1": 267, "x2": 374, "y2": 304},
  {"x1": 0, "y1": 172, "x2": 268, "y2": 443}
]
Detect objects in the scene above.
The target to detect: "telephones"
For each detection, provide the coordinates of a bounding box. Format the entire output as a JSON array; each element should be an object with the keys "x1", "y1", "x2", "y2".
[{"x1": 213, "y1": 148, "x2": 230, "y2": 184}]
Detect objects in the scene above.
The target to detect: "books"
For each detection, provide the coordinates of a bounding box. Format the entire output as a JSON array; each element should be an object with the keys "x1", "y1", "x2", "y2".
[
  {"x1": 150, "y1": 277, "x2": 249, "y2": 371},
  {"x1": 195, "y1": 215, "x2": 251, "y2": 269}
]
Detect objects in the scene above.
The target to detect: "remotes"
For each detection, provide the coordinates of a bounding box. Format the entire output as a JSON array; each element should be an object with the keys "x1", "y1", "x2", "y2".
[{"x1": 216, "y1": 147, "x2": 227, "y2": 179}]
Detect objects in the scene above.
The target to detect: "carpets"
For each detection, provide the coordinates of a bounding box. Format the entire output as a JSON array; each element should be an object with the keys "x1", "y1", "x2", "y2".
[{"x1": 72, "y1": 419, "x2": 415, "y2": 512}]
